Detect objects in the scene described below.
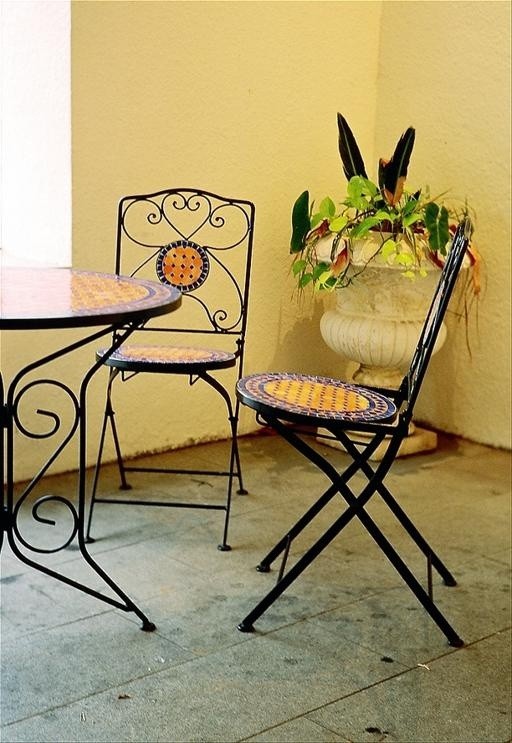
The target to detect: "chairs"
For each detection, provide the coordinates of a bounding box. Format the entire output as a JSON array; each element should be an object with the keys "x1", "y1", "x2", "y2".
[
  {"x1": 82, "y1": 186, "x2": 254, "y2": 551},
  {"x1": 235, "y1": 217, "x2": 470, "y2": 648}
]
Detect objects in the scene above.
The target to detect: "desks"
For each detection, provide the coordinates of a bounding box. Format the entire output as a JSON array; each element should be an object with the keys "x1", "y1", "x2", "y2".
[{"x1": 0, "y1": 267, "x2": 183, "y2": 631}]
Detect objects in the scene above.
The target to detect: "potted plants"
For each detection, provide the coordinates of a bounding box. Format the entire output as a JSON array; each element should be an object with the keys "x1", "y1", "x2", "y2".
[{"x1": 284, "y1": 110, "x2": 487, "y2": 461}]
